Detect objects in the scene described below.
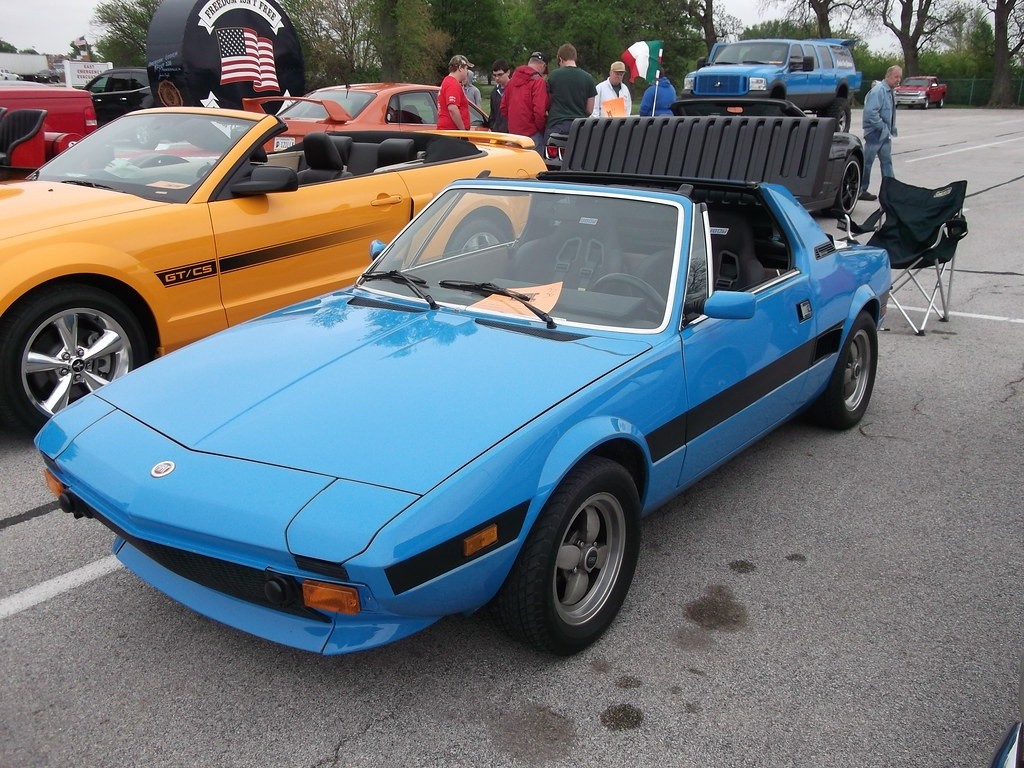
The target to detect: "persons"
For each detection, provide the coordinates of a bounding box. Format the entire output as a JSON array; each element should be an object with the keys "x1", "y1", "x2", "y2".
[
  {"x1": 437, "y1": 43, "x2": 677, "y2": 157},
  {"x1": 856, "y1": 66, "x2": 902, "y2": 200}
]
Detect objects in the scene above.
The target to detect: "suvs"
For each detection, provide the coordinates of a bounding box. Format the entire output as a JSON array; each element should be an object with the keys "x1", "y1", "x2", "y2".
[
  {"x1": 81, "y1": 66, "x2": 164, "y2": 147},
  {"x1": 682, "y1": 38, "x2": 863, "y2": 133},
  {"x1": 0, "y1": 79, "x2": 104, "y2": 178}
]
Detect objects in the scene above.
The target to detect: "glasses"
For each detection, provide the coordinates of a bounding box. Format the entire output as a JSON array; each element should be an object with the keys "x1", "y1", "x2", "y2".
[{"x1": 492, "y1": 71, "x2": 506, "y2": 77}]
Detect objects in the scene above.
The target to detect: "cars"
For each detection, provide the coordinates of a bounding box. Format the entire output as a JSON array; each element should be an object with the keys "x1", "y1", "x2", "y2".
[
  {"x1": 241, "y1": 82, "x2": 492, "y2": 152},
  {"x1": 893, "y1": 77, "x2": 947, "y2": 110},
  {"x1": 30, "y1": 115, "x2": 891, "y2": 655}
]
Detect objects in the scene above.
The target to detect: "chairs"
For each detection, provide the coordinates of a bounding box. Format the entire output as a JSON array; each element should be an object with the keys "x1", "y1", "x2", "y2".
[
  {"x1": 250, "y1": 132, "x2": 417, "y2": 184},
  {"x1": 742, "y1": 50, "x2": 763, "y2": 60},
  {"x1": 510, "y1": 223, "x2": 765, "y2": 322},
  {"x1": 403, "y1": 105, "x2": 418, "y2": 115},
  {"x1": 831, "y1": 176, "x2": 969, "y2": 336}
]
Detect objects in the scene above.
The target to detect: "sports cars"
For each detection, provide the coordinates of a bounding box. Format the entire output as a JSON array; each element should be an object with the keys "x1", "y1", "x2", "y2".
[
  {"x1": 544, "y1": 98, "x2": 866, "y2": 215},
  {"x1": 0, "y1": 104, "x2": 548, "y2": 436}
]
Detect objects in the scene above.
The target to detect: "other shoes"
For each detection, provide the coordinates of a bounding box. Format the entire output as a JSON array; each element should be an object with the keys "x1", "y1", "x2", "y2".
[{"x1": 859, "y1": 191, "x2": 878, "y2": 201}]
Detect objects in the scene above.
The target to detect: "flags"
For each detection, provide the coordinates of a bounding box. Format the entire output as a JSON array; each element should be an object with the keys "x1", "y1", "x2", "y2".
[{"x1": 622, "y1": 39, "x2": 664, "y2": 86}]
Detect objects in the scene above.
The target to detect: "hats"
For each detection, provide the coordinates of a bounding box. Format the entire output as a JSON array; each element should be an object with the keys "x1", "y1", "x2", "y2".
[
  {"x1": 449, "y1": 55, "x2": 475, "y2": 68},
  {"x1": 528, "y1": 51, "x2": 549, "y2": 75},
  {"x1": 610, "y1": 61, "x2": 626, "y2": 72}
]
